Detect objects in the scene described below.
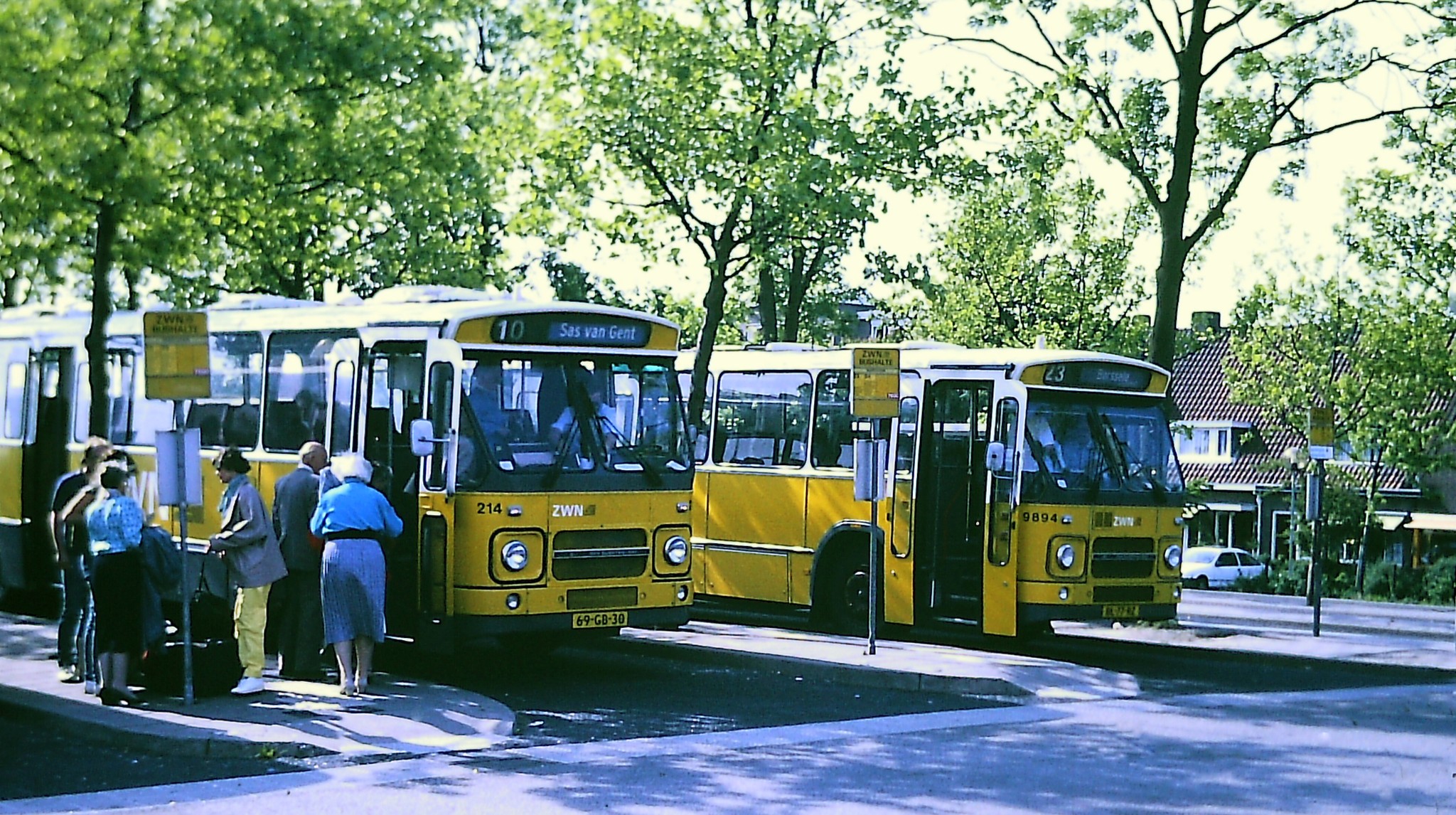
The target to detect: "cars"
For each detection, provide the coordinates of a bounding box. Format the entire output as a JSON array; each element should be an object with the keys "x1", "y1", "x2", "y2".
[{"x1": 1181, "y1": 546, "x2": 1272, "y2": 590}]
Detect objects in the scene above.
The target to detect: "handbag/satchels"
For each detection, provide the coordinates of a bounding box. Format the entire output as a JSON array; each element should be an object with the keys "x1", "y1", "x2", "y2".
[{"x1": 191, "y1": 591, "x2": 229, "y2": 628}]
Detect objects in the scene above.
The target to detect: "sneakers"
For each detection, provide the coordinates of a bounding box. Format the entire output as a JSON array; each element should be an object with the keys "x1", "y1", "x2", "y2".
[{"x1": 231, "y1": 677, "x2": 264, "y2": 694}]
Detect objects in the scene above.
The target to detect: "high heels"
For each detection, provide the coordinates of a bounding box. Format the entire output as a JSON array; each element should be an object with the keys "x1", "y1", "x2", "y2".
[
  {"x1": 340, "y1": 679, "x2": 355, "y2": 696},
  {"x1": 354, "y1": 677, "x2": 366, "y2": 694}
]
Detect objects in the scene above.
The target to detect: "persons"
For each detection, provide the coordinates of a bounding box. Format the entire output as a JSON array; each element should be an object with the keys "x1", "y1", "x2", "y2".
[
  {"x1": 310, "y1": 455, "x2": 403, "y2": 696},
  {"x1": 85, "y1": 466, "x2": 139, "y2": 706},
  {"x1": 549, "y1": 375, "x2": 618, "y2": 453},
  {"x1": 49, "y1": 434, "x2": 134, "y2": 695},
  {"x1": 470, "y1": 360, "x2": 510, "y2": 435},
  {"x1": 208, "y1": 446, "x2": 289, "y2": 694},
  {"x1": 1007, "y1": 413, "x2": 1059, "y2": 494},
  {"x1": 268, "y1": 441, "x2": 327, "y2": 683}
]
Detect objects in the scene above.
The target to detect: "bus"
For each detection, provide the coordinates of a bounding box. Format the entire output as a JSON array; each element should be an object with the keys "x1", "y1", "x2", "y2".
[
  {"x1": 0, "y1": 285, "x2": 710, "y2": 676},
  {"x1": 473, "y1": 339, "x2": 1187, "y2": 642}
]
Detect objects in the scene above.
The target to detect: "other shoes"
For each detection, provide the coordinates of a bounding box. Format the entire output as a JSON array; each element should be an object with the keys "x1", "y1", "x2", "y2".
[
  {"x1": 59, "y1": 667, "x2": 82, "y2": 683},
  {"x1": 279, "y1": 667, "x2": 327, "y2": 683},
  {"x1": 85, "y1": 681, "x2": 97, "y2": 693},
  {"x1": 95, "y1": 685, "x2": 103, "y2": 696},
  {"x1": 100, "y1": 686, "x2": 150, "y2": 706}
]
